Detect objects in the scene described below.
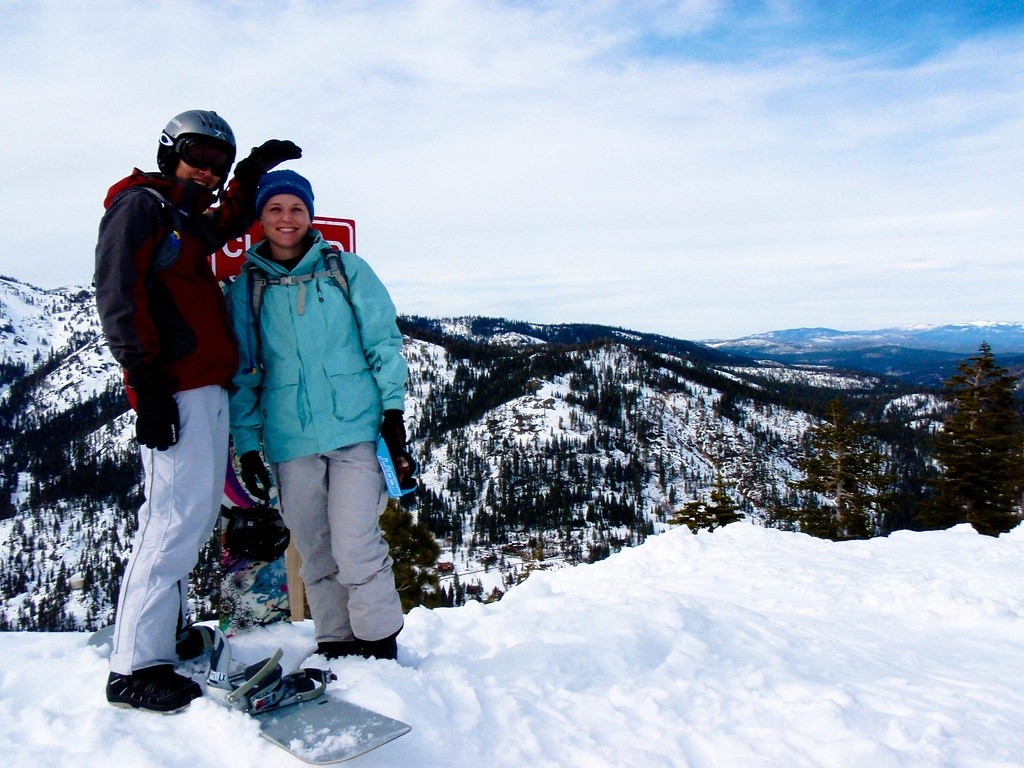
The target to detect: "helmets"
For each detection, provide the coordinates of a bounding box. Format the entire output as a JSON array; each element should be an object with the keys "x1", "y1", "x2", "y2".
[{"x1": 157, "y1": 109, "x2": 236, "y2": 191}]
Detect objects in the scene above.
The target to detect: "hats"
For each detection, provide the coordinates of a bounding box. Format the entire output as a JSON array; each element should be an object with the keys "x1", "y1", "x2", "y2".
[{"x1": 254, "y1": 169, "x2": 314, "y2": 223}]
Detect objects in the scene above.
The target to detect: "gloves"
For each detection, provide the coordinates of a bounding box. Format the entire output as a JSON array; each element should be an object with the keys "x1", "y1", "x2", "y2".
[
  {"x1": 127, "y1": 357, "x2": 181, "y2": 451},
  {"x1": 381, "y1": 410, "x2": 407, "y2": 461},
  {"x1": 233, "y1": 140, "x2": 303, "y2": 194},
  {"x1": 239, "y1": 451, "x2": 271, "y2": 499}
]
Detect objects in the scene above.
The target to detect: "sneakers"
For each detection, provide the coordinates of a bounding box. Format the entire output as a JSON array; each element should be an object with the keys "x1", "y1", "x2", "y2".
[
  {"x1": 174, "y1": 626, "x2": 215, "y2": 661},
  {"x1": 107, "y1": 666, "x2": 203, "y2": 715}
]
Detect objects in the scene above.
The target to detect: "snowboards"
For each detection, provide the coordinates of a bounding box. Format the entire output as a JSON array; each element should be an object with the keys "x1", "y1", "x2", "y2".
[
  {"x1": 85, "y1": 621, "x2": 413, "y2": 765},
  {"x1": 219, "y1": 435, "x2": 291, "y2": 638}
]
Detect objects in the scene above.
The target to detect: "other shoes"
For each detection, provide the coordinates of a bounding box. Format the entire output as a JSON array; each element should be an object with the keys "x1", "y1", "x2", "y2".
[
  {"x1": 314, "y1": 640, "x2": 356, "y2": 660},
  {"x1": 353, "y1": 624, "x2": 405, "y2": 659}
]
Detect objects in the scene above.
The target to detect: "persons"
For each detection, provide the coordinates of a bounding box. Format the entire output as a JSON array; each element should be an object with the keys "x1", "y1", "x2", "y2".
[
  {"x1": 94, "y1": 109, "x2": 302, "y2": 715},
  {"x1": 229, "y1": 169, "x2": 408, "y2": 661}
]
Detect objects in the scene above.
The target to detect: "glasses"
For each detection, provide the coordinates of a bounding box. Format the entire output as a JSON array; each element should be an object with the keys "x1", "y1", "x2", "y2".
[
  {"x1": 178, "y1": 135, "x2": 235, "y2": 178},
  {"x1": 386, "y1": 450, "x2": 417, "y2": 500}
]
